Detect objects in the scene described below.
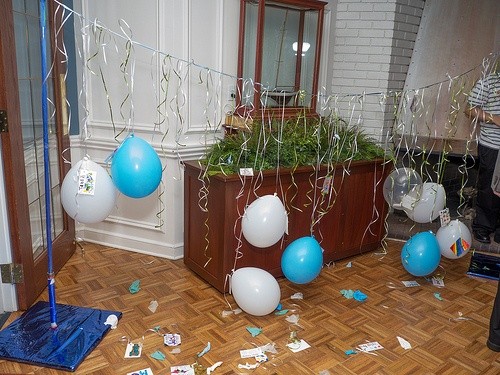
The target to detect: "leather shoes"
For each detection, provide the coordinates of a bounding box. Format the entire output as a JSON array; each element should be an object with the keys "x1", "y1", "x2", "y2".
[{"x1": 472, "y1": 228, "x2": 490, "y2": 242}]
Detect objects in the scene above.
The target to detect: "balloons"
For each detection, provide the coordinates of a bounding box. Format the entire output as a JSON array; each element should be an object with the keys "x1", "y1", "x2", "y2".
[
  {"x1": 383, "y1": 168, "x2": 423, "y2": 210},
  {"x1": 436, "y1": 219, "x2": 472, "y2": 259},
  {"x1": 111, "y1": 134, "x2": 162, "y2": 198},
  {"x1": 61, "y1": 157, "x2": 116, "y2": 224},
  {"x1": 401, "y1": 230, "x2": 441, "y2": 276},
  {"x1": 404, "y1": 183, "x2": 446, "y2": 223},
  {"x1": 242, "y1": 192, "x2": 287, "y2": 247},
  {"x1": 229, "y1": 267, "x2": 281, "y2": 316},
  {"x1": 281, "y1": 235, "x2": 323, "y2": 284}
]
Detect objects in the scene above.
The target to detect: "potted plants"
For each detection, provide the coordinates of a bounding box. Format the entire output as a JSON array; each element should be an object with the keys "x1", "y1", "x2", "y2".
[{"x1": 178, "y1": 114, "x2": 394, "y2": 294}]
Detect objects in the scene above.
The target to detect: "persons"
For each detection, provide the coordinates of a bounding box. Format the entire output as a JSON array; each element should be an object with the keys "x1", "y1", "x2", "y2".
[
  {"x1": 487, "y1": 277, "x2": 500, "y2": 352},
  {"x1": 465, "y1": 72, "x2": 500, "y2": 243}
]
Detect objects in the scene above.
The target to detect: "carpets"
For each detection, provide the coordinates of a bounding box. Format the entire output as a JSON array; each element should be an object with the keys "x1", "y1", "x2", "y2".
[{"x1": 467, "y1": 253, "x2": 500, "y2": 279}]
